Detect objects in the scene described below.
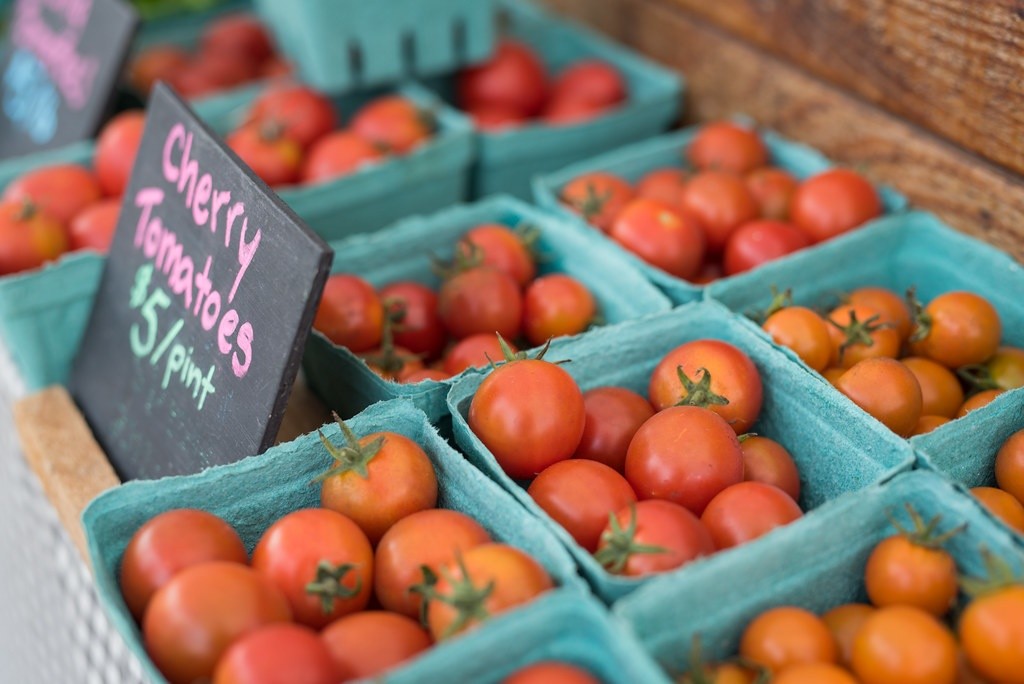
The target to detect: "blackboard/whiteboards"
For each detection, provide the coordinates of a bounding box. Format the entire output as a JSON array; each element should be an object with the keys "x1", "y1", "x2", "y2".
[{"x1": 67, "y1": 80, "x2": 337, "y2": 487}]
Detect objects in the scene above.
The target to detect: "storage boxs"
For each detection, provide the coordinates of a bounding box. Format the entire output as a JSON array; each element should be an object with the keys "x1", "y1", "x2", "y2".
[{"x1": 0, "y1": 0, "x2": 1024, "y2": 684}]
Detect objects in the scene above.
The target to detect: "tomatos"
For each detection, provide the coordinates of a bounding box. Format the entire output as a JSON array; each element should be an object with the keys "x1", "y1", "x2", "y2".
[{"x1": 0, "y1": 18, "x2": 1024, "y2": 684}]
